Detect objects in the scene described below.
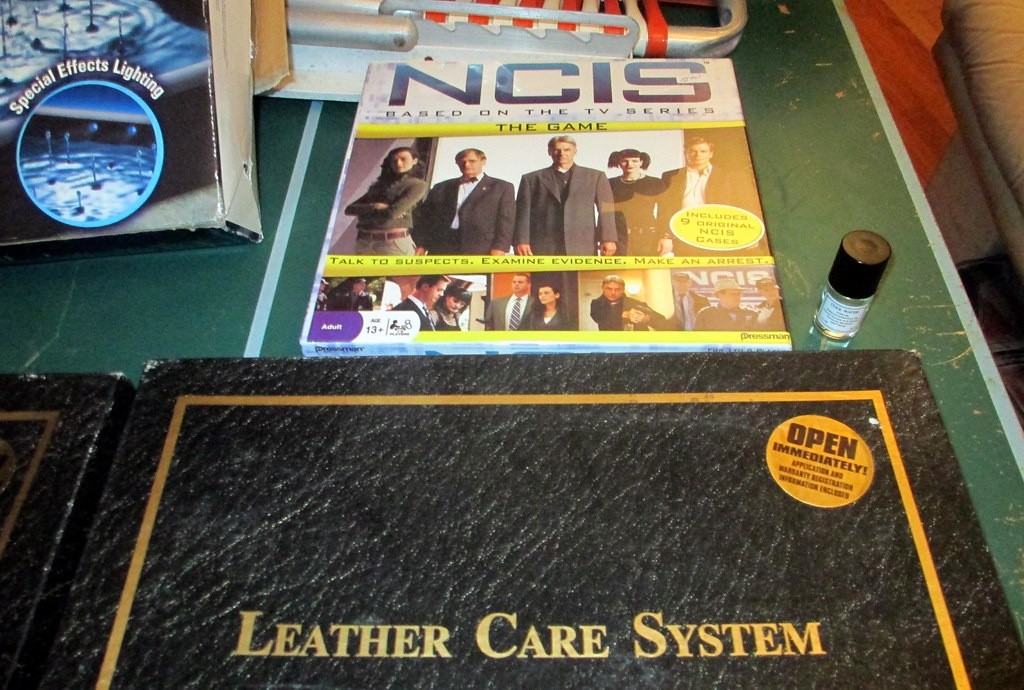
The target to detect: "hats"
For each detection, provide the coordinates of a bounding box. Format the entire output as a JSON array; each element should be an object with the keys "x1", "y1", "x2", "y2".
[
  {"x1": 713, "y1": 279, "x2": 747, "y2": 293},
  {"x1": 755, "y1": 277, "x2": 778, "y2": 288}
]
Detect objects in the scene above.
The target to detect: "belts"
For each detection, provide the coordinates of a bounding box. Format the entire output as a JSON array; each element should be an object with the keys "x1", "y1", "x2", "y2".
[{"x1": 357, "y1": 231, "x2": 409, "y2": 240}]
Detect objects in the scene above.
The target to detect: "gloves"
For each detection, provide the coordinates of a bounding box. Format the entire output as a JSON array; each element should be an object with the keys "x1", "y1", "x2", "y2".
[{"x1": 757, "y1": 307, "x2": 774, "y2": 321}]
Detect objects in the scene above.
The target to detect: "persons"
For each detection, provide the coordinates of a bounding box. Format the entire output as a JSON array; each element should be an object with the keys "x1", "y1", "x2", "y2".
[{"x1": 316, "y1": 123, "x2": 782, "y2": 333}]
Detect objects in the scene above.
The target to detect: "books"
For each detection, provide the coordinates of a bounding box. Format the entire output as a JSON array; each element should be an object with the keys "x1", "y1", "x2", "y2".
[{"x1": 300, "y1": 51, "x2": 793, "y2": 359}]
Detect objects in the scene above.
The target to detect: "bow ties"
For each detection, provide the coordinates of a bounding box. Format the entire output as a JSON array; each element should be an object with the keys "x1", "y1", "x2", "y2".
[{"x1": 460, "y1": 177, "x2": 479, "y2": 184}]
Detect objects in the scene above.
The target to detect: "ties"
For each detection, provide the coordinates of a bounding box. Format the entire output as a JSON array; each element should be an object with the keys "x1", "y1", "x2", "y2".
[
  {"x1": 423, "y1": 306, "x2": 435, "y2": 331},
  {"x1": 678, "y1": 294, "x2": 687, "y2": 328},
  {"x1": 508, "y1": 298, "x2": 522, "y2": 331}
]
